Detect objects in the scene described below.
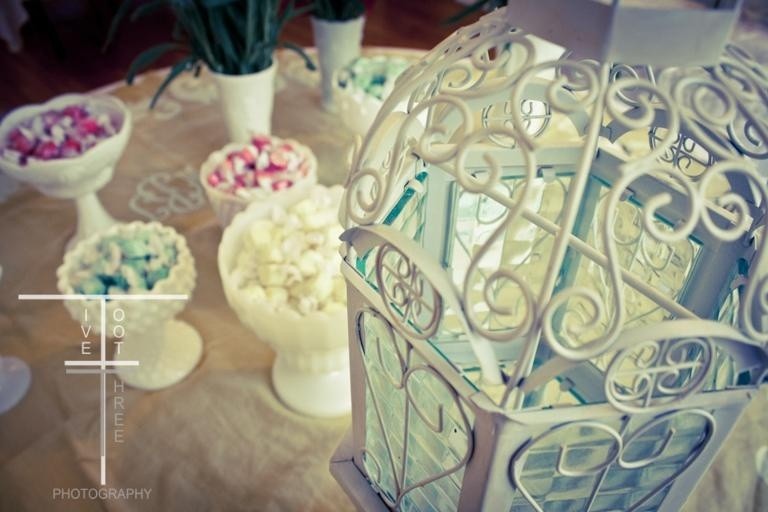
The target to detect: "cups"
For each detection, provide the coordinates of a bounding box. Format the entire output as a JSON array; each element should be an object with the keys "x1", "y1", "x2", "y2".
[{"x1": 198, "y1": 134, "x2": 316, "y2": 228}]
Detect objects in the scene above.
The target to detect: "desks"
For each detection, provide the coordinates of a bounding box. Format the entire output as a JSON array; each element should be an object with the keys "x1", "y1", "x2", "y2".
[{"x1": 0, "y1": 45, "x2": 768, "y2": 511}]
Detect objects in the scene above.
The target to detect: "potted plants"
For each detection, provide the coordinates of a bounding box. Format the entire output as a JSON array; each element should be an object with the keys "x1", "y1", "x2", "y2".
[
  {"x1": 306, "y1": 0, "x2": 368, "y2": 113},
  {"x1": 97, "y1": 0, "x2": 320, "y2": 149}
]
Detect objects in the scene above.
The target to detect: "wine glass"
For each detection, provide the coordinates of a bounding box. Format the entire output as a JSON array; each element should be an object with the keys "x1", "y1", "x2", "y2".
[
  {"x1": 331, "y1": 50, "x2": 416, "y2": 174},
  {"x1": 0, "y1": 90, "x2": 132, "y2": 260},
  {"x1": 55, "y1": 220, "x2": 204, "y2": 393},
  {"x1": 216, "y1": 184, "x2": 355, "y2": 415}
]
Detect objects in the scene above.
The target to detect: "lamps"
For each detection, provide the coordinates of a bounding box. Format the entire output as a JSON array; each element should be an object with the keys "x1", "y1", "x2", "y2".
[{"x1": 326, "y1": 0, "x2": 768, "y2": 510}]
